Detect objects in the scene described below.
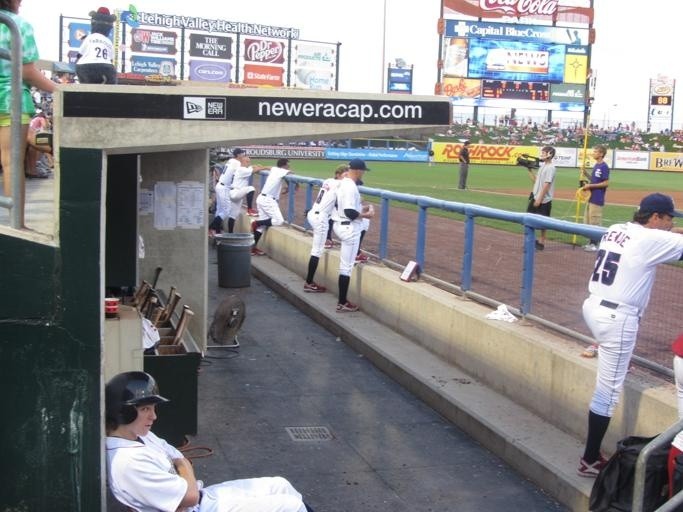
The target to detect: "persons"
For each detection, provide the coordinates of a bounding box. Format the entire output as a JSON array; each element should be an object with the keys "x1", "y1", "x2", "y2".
[
  {"x1": 102, "y1": 371, "x2": 309, "y2": 512},
  {"x1": 581, "y1": 144, "x2": 611, "y2": 253},
  {"x1": 207, "y1": 148, "x2": 295, "y2": 257},
  {"x1": 526, "y1": 146, "x2": 556, "y2": 250},
  {"x1": 442, "y1": 109, "x2": 682, "y2": 151},
  {"x1": 23, "y1": 71, "x2": 74, "y2": 179},
  {"x1": 577, "y1": 193, "x2": 682, "y2": 480},
  {"x1": 303, "y1": 164, "x2": 352, "y2": 295},
  {"x1": 331, "y1": 157, "x2": 375, "y2": 312},
  {"x1": 0, "y1": 1, "x2": 60, "y2": 231},
  {"x1": 457, "y1": 141, "x2": 471, "y2": 190}
]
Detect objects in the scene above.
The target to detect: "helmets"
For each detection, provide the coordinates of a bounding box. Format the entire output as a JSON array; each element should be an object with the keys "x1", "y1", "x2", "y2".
[{"x1": 105, "y1": 371, "x2": 171, "y2": 416}]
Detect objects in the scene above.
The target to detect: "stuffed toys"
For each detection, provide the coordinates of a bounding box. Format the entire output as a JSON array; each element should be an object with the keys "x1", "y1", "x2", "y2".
[{"x1": 73, "y1": 8, "x2": 117, "y2": 84}]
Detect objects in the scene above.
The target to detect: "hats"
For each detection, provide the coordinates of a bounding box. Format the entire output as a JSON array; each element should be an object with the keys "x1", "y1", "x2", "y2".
[
  {"x1": 348, "y1": 158, "x2": 372, "y2": 171},
  {"x1": 232, "y1": 148, "x2": 246, "y2": 155},
  {"x1": 637, "y1": 191, "x2": 683, "y2": 218},
  {"x1": 89, "y1": 6, "x2": 118, "y2": 24}
]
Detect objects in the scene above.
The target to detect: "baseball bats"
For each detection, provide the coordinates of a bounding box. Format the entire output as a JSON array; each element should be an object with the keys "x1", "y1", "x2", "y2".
[{"x1": 134, "y1": 266, "x2": 194, "y2": 345}]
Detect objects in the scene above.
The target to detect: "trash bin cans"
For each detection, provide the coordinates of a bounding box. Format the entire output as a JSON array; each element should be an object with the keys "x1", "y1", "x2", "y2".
[{"x1": 215, "y1": 233, "x2": 255, "y2": 288}]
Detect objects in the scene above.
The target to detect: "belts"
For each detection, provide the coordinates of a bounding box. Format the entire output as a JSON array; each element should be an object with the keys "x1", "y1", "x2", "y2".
[
  {"x1": 220, "y1": 183, "x2": 225, "y2": 186},
  {"x1": 599, "y1": 298, "x2": 641, "y2": 316},
  {"x1": 230, "y1": 188, "x2": 234, "y2": 190},
  {"x1": 314, "y1": 211, "x2": 320, "y2": 215},
  {"x1": 340, "y1": 221, "x2": 351, "y2": 226},
  {"x1": 261, "y1": 193, "x2": 277, "y2": 201}
]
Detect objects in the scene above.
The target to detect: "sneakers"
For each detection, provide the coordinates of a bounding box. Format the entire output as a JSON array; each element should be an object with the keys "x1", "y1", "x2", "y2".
[
  {"x1": 303, "y1": 281, "x2": 328, "y2": 293},
  {"x1": 246, "y1": 208, "x2": 259, "y2": 217},
  {"x1": 324, "y1": 239, "x2": 337, "y2": 248},
  {"x1": 535, "y1": 240, "x2": 544, "y2": 251},
  {"x1": 584, "y1": 243, "x2": 597, "y2": 252},
  {"x1": 208, "y1": 227, "x2": 224, "y2": 247},
  {"x1": 354, "y1": 253, "x2": 371, "y2": 264},
  {"x1": 576, "y1": 452, "x2": 609, "y2": 478},
  {"x1": 250, "y1": 219, "x2": 260, "y2": 233},
  {"x1": 581, "y1": 244, "x2": 591, "y2": 249},
  {"x1": 249, "y1": 247, "x2": 266, "y2": 257},
  {"x1": 336, "y1": 300, "x2": 361, "y2": 313}
]
